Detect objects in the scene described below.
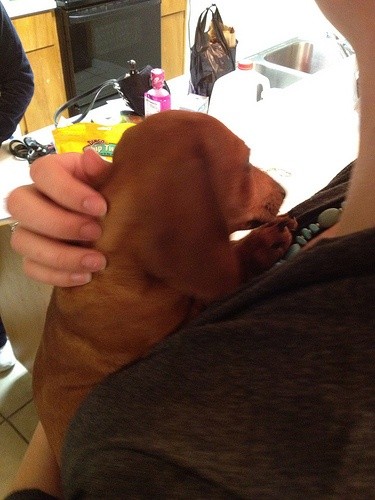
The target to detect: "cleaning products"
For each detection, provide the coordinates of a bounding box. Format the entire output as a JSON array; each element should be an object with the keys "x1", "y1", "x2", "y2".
[
  {"x1": 208, "y1": 58, "x2": 281, "y2": 127},
  {"x1": 144, "y1": 68, "x2": 171, "y2": 117}
]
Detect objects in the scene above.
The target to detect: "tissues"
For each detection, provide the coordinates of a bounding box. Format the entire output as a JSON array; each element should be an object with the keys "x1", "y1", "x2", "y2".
[{"x1": 51, "y1": 114, "x2": 137, "y2": 162}]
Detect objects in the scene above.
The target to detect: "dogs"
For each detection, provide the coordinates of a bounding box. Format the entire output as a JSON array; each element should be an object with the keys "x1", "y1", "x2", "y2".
[{"x1": 30, "y1": 111, "x2": 298, "y2": 467}]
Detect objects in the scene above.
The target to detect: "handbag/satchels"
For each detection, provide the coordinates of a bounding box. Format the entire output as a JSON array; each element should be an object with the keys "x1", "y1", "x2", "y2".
[
  {"x1": 189, "y1": 3, "x2": 238, "y2": 97},
  {"x1": 54, "y1": 65, "x2": 170, "y2": 129}
]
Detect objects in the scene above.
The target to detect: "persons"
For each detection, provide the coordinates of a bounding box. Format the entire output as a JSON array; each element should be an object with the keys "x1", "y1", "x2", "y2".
[
  {"x1": 0, "y1": 1, "x2": 35, "y2": 373},
  {"x1": 6, "y1": 0, "x2": 375, "y2": 500}
]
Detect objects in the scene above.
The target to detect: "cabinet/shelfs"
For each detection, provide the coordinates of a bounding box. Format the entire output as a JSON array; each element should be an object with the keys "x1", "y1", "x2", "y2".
[
  {"x1": 9, "y1": 10, "x2": 70, "y2": 136},
  {"x1": 160, "y1": 0, "x2": 186, "y2": 82}
]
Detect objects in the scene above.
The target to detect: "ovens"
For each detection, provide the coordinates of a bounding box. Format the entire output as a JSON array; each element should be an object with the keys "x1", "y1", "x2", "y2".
[{"x1": 52, "y1": 0, "x2": 163, "y2": 117}]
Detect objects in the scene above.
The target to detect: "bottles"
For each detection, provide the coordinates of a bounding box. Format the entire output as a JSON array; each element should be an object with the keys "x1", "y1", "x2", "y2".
[
  {"x1": 144, "y1": 68, "x2": 171, "y2": 118},
  {"x1": 207, "y1": 60, "x2": 270, "y2": 146}
]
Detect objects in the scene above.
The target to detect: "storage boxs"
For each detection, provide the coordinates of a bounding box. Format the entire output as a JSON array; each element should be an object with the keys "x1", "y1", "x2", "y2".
[{"x1": 179, "y1": 93, "x2": 209, "y2": 114}]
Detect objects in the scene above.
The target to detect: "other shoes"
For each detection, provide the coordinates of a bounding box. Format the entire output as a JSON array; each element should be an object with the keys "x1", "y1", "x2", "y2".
[{"x1": 0, "y1": 348, "x2": 17, "y2": 372}]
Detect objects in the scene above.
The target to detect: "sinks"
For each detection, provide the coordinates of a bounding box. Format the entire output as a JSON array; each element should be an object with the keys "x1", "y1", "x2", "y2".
[
  {"x1": 264, "y1": 39, "x2": 344, "y2": 73},
  {"x1": 253, "y1": 65, "x2": 298, "y2": 101}
]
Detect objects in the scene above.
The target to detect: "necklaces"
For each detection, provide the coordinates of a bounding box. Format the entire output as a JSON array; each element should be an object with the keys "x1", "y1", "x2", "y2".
[{"x1": 269, "y1": 207, "x2": 350, "y2": 273}]
